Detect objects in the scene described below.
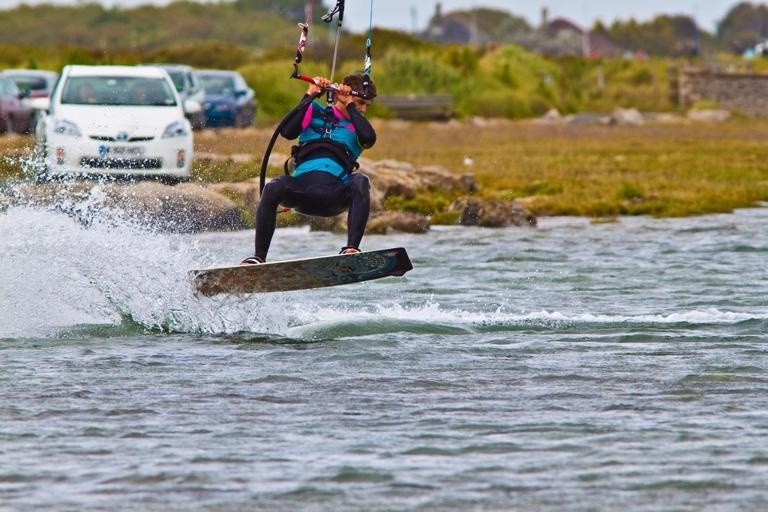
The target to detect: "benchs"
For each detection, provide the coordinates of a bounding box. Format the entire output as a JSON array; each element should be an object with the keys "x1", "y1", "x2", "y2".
[{"x1": 374, "y1": 93, "x2": 454, "y2": 123}]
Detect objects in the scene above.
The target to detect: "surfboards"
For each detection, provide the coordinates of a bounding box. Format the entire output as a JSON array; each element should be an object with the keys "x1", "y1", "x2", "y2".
[{"x1": 189, "y1": 246, "x2": 414, "y2": 297}]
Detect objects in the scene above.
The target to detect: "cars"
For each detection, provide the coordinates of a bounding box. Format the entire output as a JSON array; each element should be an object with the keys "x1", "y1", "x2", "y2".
[{"x1": 1, "y1": 63, "x2": 256, "y2": 184}]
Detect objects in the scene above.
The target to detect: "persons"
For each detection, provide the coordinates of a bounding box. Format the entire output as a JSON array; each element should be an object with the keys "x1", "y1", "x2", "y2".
[
  {"x1": 78, "y1": 81, "x2": 95, "y2": 102},
  {"x1": 131, "y1": 83, "x2": 149, "y2": 104},
  {"x1": 239, "y1": 74, "x2": 377, "y2": 265}
]
